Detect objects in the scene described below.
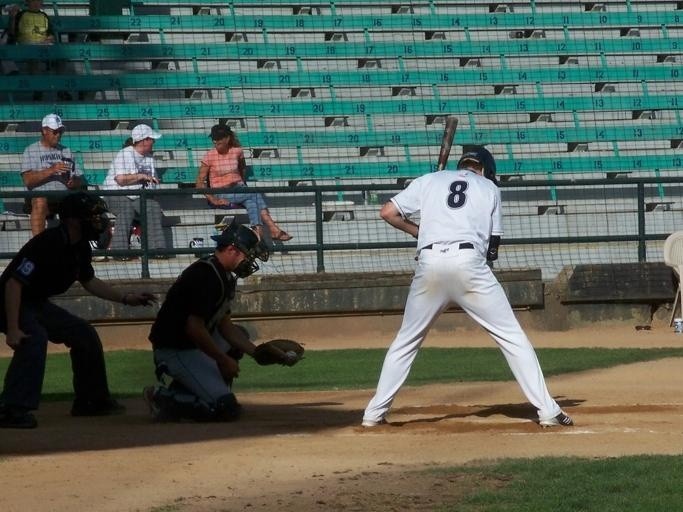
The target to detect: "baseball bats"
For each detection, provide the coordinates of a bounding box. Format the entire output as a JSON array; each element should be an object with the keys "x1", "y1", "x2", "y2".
[{"x1": 436, "y1": 118, "x2": 459, "y2": 171}]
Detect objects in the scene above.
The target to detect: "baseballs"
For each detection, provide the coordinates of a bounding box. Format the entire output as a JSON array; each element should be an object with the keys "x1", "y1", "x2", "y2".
[{"x1": 286, "y1": 351, "x2": 296, "y2": 358}]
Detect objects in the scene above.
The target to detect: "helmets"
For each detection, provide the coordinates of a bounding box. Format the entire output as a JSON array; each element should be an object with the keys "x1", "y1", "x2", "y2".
[
  {"x1": 59, "y1": 192, "x2": 117, "y2": 250},
  {"x1": 210, "y1": 221, "x2": 270, "y2": 279},
  {"x1": 456, "y1": 145, "x2": 499, "y2": 185}
]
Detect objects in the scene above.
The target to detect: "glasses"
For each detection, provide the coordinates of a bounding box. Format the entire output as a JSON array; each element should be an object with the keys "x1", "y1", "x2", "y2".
[{"x1": 46, "y1": 128, "x2": 63, "y2": 136}]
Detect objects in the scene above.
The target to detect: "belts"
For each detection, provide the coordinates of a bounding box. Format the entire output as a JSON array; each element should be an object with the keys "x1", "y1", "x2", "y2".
[{"x1": 420, "y1": 242, "x2": 478, "y2": 250}]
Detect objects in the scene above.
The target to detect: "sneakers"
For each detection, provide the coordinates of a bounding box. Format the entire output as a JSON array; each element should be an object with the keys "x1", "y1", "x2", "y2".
[
  {"x1": 540, "y1": 409, "x2": 574, "y2": 428},
  {"x1": 0, "y1": 410, "x2": 39, "y2": 429},
  {"x1": 360, "y1": 418, "x2": 388, "y2": 429},
  {"x1": 142, "y1": 385, "x2": 179, "y2": 424},
  {"x1": 71, "y1": 401, "x2": 126, "y2": 419}
]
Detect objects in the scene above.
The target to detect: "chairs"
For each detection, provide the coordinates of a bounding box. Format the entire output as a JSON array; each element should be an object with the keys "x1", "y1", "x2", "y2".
[{"x1": 660, "y1": 231, "x2": 682, "y2": 326}]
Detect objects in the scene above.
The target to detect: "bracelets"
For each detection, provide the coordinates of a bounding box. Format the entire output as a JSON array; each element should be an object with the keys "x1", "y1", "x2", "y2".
[{"x1": 122, "y1": 291, "x2": 136, "y2": 306}]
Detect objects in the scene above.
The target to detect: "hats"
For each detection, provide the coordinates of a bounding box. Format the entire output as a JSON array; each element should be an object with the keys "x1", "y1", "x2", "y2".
[
  {"x1": 41, "y1": 113, "x2": 65, "y2": 130},
  {"x1": 209, "y1": 124, "x2": 231, "y2": 139},
  {"x1": 131, "y1": 123, "x2": 162, "y2": 145}
]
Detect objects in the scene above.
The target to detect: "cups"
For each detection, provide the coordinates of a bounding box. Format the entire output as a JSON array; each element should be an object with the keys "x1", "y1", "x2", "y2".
[
  {"x1": 370, "y1": 191, "x2": 379, "y2": 204},
  {"x1": 193, "y1": 237, "x2": 203, "y2": 248},
  {"x1": 673, "y1": 318, "x2": 683, "y2": 333},
  {"x1": 147, "y1": 176, "x2": 155, "y2": 190},
  {"x1": 61, "y1": 160, "x2": 73, "y2": 180}
]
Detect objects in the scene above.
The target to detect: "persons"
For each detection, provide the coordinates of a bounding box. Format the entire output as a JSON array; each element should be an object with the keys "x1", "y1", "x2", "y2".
[
  {"x1": 196, "y1": 124, "x2": 292, "y2": 248},
  {"x1": 360, "y1": 145, "x2": 575, "y2": 428},
  {"x1": 0, "y1": 193, "x2": 158, "y2": 429},
  {"x1": 8, "y1": 0, "x2": 73, "y2": 102},
  {"x1": 143, "y1": 224, "x2": 300, "y2": 423},
  {"x1": 20, "y1": 114, "x2": 84, "y2": 237},
  {"x1": 98, "y1": 123, "x2": 170, "y2": 260}
]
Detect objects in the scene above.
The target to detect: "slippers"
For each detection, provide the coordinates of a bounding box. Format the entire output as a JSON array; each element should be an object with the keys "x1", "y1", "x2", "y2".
[{"x1": 271, "y1": 228, "x2": 293, "y2": 241}]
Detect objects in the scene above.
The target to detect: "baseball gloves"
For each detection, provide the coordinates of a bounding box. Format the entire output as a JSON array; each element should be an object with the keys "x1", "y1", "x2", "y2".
[{"x1": 255, "y1": 339, "x2": 305, "y2": 366}]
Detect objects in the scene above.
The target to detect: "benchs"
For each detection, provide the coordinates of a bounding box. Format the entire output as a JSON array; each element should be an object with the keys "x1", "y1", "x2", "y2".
[{"x1": 0, "y1": 3, "x2": 683, "y2": 233}]
